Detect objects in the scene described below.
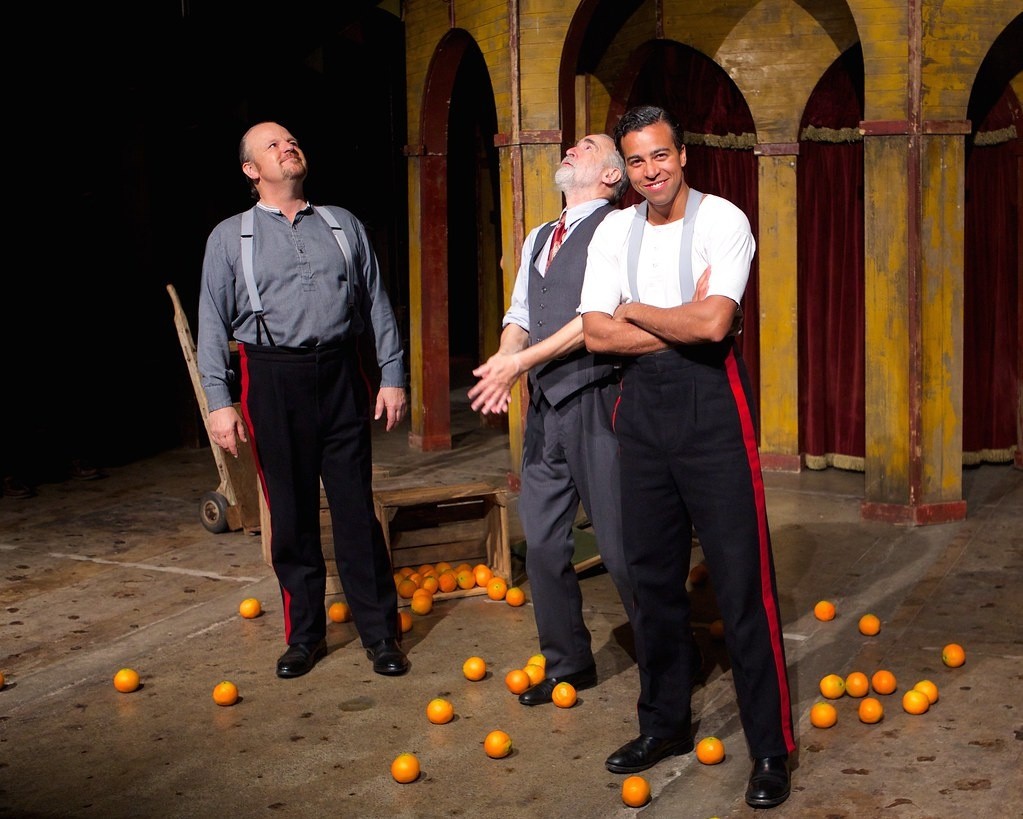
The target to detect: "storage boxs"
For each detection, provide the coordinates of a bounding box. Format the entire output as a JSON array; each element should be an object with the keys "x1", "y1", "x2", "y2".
[{"x1": 373, "y1": 482, "x2": 512, "y2": 606}]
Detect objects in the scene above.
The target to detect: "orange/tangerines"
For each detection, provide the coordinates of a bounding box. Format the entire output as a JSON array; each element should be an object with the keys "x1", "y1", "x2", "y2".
[{"x1": 114, "y1": 563, "x2": 968, "y2": 806}]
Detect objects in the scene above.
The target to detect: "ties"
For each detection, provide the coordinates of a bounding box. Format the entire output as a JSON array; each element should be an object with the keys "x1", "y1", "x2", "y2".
[{"x1": 545, "y1": 211, "x2": 567, "y2": 270}]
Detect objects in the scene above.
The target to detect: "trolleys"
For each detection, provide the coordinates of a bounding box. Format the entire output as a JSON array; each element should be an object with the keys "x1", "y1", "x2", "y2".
[{"x1": 166, "y1": 284, "x2": 261, "y2": 536}]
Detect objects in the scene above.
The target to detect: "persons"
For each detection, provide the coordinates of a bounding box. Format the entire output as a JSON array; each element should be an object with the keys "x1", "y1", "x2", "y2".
[
  {"x1": 576, "y1": 102, "x2": 799, "y2": 808},
  {"x1": 466, "y1": 133, "x2": 637, "y2": 706},
  {"x1": 198, "y1": 120, "x2": 409, "y2": 679}
]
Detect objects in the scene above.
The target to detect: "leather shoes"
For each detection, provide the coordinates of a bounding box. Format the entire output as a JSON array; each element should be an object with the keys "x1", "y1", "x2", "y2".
[
  {"x1": 275, "y1": 635, "x2": 328, "y2": 676},
  {"x1": 367, "y1": 639, "x2": 409, "y2": 674},
  {"x1": 519, "y1": 665, "x2": 597, "y2": 705},
  {"x1": 603, "y1": 732, "x2": 694, "y2": 773},
  {"x1": 745, "y1": 753, "x2": 791, "y2": 806}
]
